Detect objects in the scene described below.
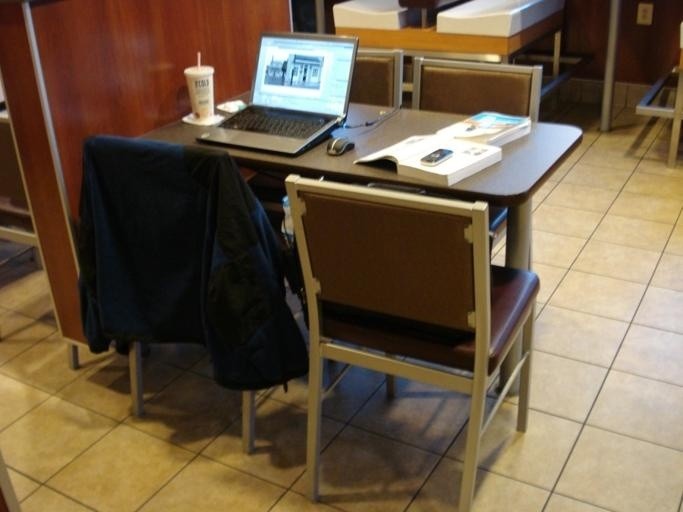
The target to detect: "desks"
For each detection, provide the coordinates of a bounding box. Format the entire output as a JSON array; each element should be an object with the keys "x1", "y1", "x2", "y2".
[{"x1": 136, "y1": 92, "x2": 585, "y2": 393}]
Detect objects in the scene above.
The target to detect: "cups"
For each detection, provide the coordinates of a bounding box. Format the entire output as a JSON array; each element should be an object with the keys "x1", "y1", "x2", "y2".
[{"x1": 183, "y1": 65, "x2": 215, "y2": 123}]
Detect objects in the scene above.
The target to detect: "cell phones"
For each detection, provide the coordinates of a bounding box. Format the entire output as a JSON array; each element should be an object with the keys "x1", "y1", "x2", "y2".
[{"x1": 421, "y1": 149, "x2": 455, "y2": 166}]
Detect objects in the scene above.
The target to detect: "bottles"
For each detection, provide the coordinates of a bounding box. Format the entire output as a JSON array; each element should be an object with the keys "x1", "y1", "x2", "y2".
[{"x1": 282, "y1": 195, "x2": 295, "y2": 248}]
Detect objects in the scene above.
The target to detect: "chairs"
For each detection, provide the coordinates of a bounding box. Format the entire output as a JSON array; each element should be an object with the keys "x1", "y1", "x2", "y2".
[
  {"x1": 283, "y1": 173, "x2": 539, "y2": 512},
  {"x1": 411, "y1": 55, "x2": 543, "y2": 122},
  {"x1": 77, "y1": 136, "x2": 301, "y2": 456},
  {"x1": 349, "y1": 50, "x2": 406, "y2": 106}
]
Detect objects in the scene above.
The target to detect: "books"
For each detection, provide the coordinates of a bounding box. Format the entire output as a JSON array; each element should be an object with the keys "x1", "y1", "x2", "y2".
[
  {"x1": 434, "y1": 110, "x2": 531, "y2": 148},
  {"x1": 352, "y1": 133, "x2": 503, "y2": 187}
]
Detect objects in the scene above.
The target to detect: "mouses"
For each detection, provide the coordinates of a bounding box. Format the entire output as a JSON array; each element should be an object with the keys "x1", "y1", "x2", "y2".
[{"x1": 328, "y1": 136, "x2": 353, "y2": 156}]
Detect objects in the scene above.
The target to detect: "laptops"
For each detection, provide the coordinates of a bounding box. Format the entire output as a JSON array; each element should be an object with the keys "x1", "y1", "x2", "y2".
[{"x1": 193, "y1": 30, "x2": 360, "y2": 157}]
